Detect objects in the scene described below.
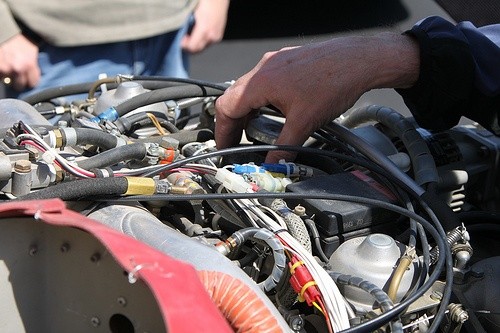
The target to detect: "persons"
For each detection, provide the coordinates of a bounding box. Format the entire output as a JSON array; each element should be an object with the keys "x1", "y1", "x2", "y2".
[
  {"x1": 215, "y1": 16, "x2": 499, "y2": 333},
  {"x1": 1, "y1": 1, "x2": 231, "y2": 126}
]
